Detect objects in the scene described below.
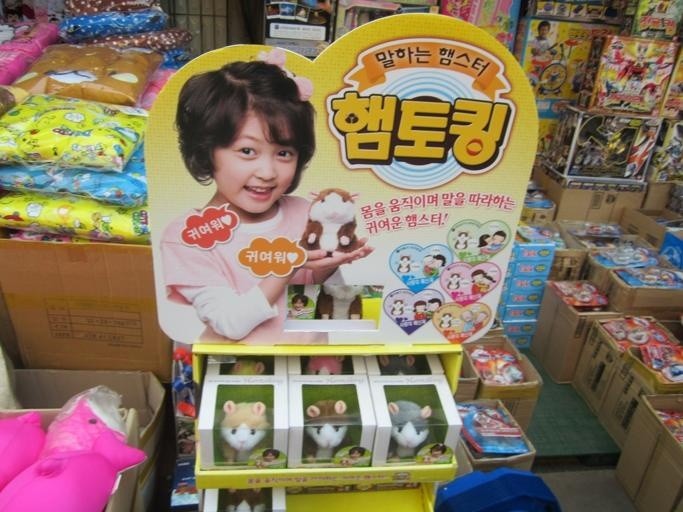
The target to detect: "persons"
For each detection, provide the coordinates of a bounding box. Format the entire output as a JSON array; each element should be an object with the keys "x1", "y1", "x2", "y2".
[
  {"x1": 160, "y1": 62, "x2": 374, "y2": 341},
  {"x1": 288, "y1": 294, "x2": 313, "y2": 319}
]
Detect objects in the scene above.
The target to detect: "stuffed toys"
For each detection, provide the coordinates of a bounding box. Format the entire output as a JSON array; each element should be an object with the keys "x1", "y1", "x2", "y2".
[
  {"x1": 0, "y1": 385, "x2": 147, "y2": 512},
  {"x1": 216, "y1": 355, "x2": 433, "y2": 512}
]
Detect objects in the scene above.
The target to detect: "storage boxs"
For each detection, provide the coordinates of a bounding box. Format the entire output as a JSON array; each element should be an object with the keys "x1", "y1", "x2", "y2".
[
  {"x1": 259, "y1": 2, "x2": 451, "y2": 56},
  {"x1": 448, "y1": 396, "x2": 537, "y2": 473},
  {"x1": 1, "y1": 367, "x2": 166, "y2": 507},
  {"x1": 453, "y1": 348, "x2": 475, "y2": 398},
  {"x1": 477, "y1": 332, "x2": 540, "y2": 432},
  {"x1": 595, "y1": 347, "x2": 681, "y2": 448},
  {"x1": 192, "y1": 350, "x2": 462, "y2": 512},
  {"x1": 0, "y1": 407, "x2": 135, "y2": 512},
  {"x1": 527, "y1": 278, "x2": 621, "y2": 387},
  {"x1": 572, "y1": 314, "x2": 673, "y2": 413},
  {"x1": 456, "y1": 0, "x2": 680, "y2": 348},
  {"x1": 1, "y1": 239, "x2": 172, "y2": 383},
  {"x1": 614, "y1": 394, "x2": 681, "y2": 511}
]
